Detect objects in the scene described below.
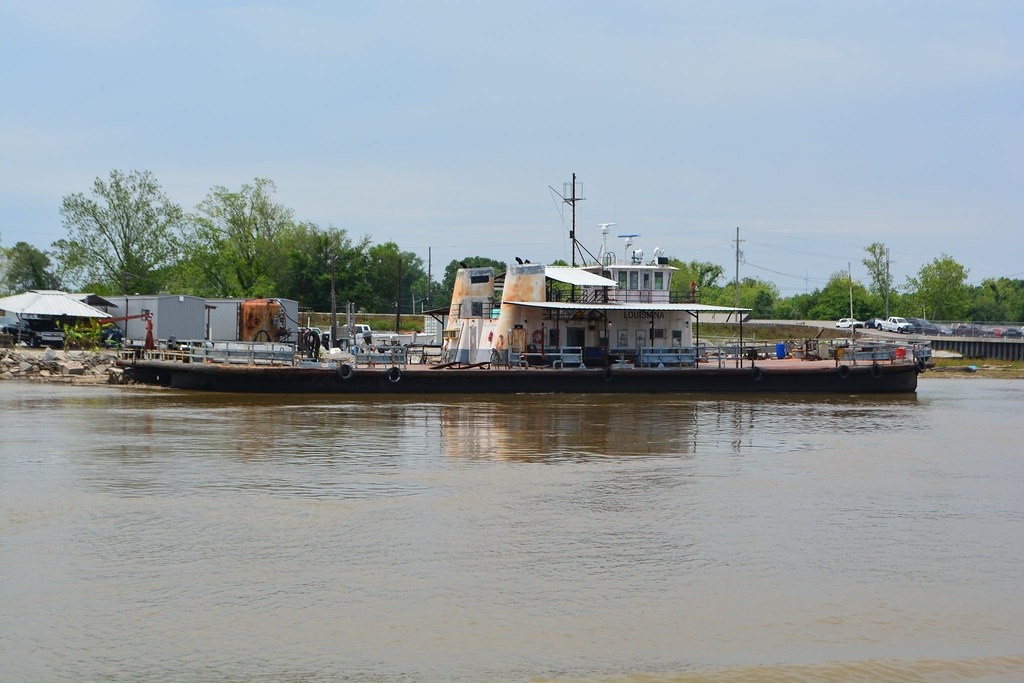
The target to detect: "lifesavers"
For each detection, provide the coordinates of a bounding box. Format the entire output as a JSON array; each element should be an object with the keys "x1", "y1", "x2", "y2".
[
  {"x1": 386, "y1": 368, "x2": 401, "y2": 384},
  {"x1": 751, "y1": 367, "x2": 762, "y2": 381},
  {"x1": 533, "y1": 330, "x2": 543, "y2": 344},
  {"x1": 338, "y1": 364, "x2": 352, "y2": 380},
  {"x1": 837, "y1": 366, "x2": 850, "y2": 378},
  {"x1": 871, "y1": 366, "x2": 881, "y2": 380},
  {"x1": 916, "y1": 361, "x2": 926, "y2": 373}
]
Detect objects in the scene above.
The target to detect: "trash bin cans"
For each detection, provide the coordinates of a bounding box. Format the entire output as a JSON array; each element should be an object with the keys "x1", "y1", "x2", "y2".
[{"x1": 776, "y1": 343, "x2": 785, "y2": 359}]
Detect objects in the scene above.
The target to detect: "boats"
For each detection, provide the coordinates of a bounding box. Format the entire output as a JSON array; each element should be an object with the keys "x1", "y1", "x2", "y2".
[{"x1": 113, "y1": 172, "x2": 933, "y2": 391}]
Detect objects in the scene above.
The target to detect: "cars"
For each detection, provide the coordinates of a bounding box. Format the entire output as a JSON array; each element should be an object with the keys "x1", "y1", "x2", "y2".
[
  {"x1": 906, "y1": 318, "x2": 1024, "y2": 339},
  {"x1": 836, "y1": 317, "x2": 863, "y2": 329},
  {"x1": 864, "y1": 318, "x2": 876, "y2": 329}
]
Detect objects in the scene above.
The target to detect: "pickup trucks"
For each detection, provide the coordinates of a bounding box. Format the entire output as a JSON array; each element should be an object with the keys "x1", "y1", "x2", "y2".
[
  {"x1": 0, "y1": 318, "x2": 66, "y2": 349},
  {"x1": 874, "y1": 316, "x2": 915, "y2": 334}
]
